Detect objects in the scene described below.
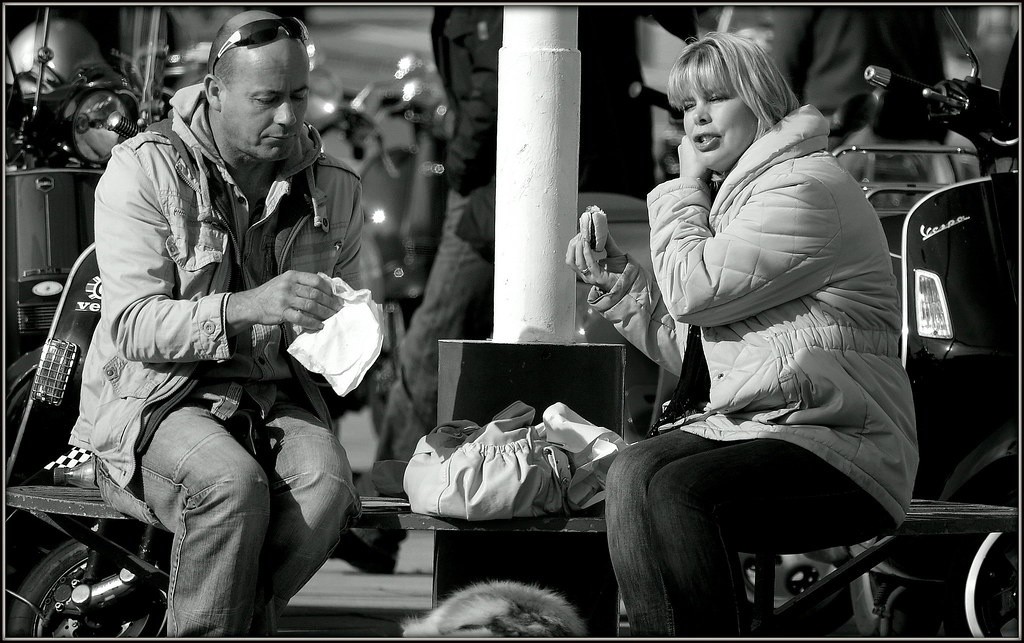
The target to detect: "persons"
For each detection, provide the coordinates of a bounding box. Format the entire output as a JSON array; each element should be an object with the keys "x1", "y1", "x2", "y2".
[
  {"x1": 69, "y1": 10, "x2": 368, "y2": 638},
  {"x1": 371, "y1": 7, "x2": 729, "y2": 493},
  {"x1": 568, "y1": 32, "x2": 919, "y2": 635},
  {"x1": 402, "y1": 581, "x2": 586, "y2": 637},
  {"x1": 768, "y1": 7, "x2": 885, "y2": 156}
]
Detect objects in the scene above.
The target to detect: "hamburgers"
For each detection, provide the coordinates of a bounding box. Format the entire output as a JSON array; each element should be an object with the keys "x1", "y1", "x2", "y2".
[{"x1": 579, "y1": 205, "x2": 607, "y2": 252}]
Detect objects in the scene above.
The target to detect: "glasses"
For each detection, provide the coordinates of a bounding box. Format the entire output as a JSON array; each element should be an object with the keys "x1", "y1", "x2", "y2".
[{"x1": 212, "y1": 16, "x2": 309, "y2": 77}]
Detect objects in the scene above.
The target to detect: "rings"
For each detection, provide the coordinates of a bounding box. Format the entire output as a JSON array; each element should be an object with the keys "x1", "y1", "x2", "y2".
[{"x1": 581, "y1": 268, "x2": 590, "y2": 275}]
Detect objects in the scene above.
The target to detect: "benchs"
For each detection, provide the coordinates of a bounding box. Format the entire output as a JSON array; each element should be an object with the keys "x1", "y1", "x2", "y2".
[{"x1": 5, "y1": 483, "x2": 1019, "y2": 637}]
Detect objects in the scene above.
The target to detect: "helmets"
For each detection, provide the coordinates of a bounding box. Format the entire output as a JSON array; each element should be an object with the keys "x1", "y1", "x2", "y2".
[{"x1": 7, "y1": 16, "x2": 107, "y2": 102}]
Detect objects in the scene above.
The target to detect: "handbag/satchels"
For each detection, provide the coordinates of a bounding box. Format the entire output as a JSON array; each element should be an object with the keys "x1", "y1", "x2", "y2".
[{"x1": 403, "y1": 402, "x2": 573, "y2": 520}]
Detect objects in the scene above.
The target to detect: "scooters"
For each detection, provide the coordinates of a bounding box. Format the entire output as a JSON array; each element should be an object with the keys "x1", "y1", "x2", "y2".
[
  {"x1": 304, "y1": 52, "x2": 466, "y2": 429},
  {"x1": 812, "y1": 8, "x2": 1024, "y2": 642}
]
